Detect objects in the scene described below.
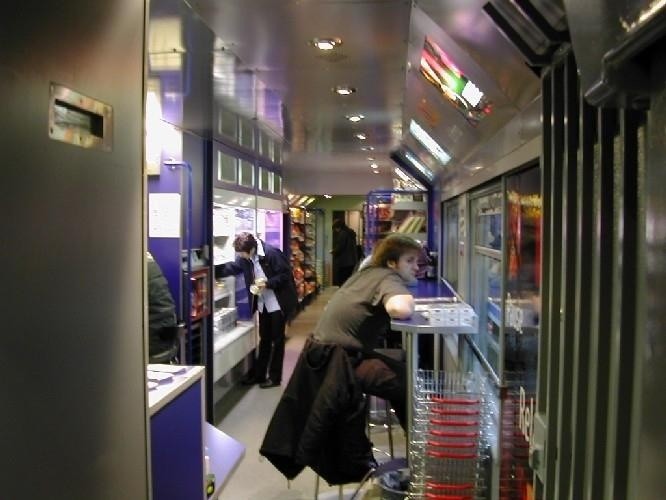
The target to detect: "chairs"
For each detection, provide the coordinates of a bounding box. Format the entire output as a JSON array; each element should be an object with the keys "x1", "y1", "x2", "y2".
[{"x1": 301, "y1": 332, "x2": 393, "y2": 500}]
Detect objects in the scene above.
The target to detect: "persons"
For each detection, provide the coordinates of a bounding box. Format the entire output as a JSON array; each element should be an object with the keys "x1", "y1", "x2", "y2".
[
  {"x1": 147, "y1": 251, "x2": 178, "y2": 356},
  {"x1": 216, "y1": 233, "x2": 300, "y2": 387},
  {"x1": 258, "y1": 234, "x2": 422, "y2": 486},
  {"x1": 328, "y1": 219, "x2": 359, "y2": 288}
]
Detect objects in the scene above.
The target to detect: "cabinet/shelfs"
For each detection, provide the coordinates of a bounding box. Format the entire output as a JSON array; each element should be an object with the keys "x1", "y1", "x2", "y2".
[
  {"x1": 467, "y1": 186, "x2": 545, "y2": 477},
  {"x1": 366, "y1": 189, "x2": 432, "y2": 278},
  {"x1": 212, "y1": 189, "x2": 284, "y2": 405},
  {"x1": 285, "y1": 206, "x2": 320, "y2": 304},
  {"x1": 438, "y1": 203, "x2": 470, "y2": 313}
]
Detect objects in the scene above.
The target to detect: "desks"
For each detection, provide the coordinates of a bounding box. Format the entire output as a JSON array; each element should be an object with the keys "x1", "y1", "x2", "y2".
[{"x1": 383, "y1": 276, "x2": 476, "y2": 494}]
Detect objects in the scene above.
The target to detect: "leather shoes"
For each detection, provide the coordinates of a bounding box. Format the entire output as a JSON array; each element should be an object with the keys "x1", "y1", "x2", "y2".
[
  {"x1": 243, "y1": 374, "x2": 265, "y2": 385},
  {"x1": 260, "y1": 378, "x2": 281, "y2": 388}
]
